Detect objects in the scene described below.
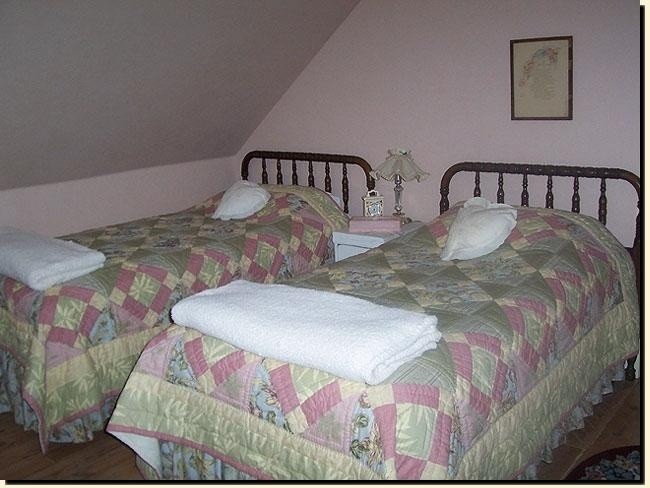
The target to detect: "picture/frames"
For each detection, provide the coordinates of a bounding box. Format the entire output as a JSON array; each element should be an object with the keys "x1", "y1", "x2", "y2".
[{"x1": 511, "y1": 35, "x2": 573, "y2": 122}]
